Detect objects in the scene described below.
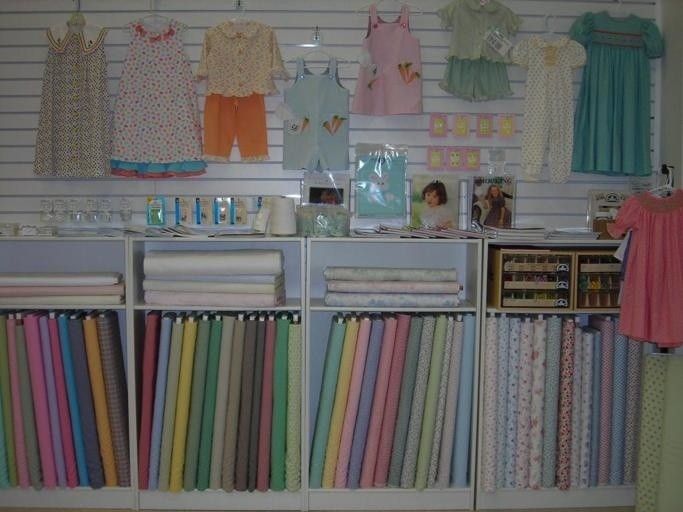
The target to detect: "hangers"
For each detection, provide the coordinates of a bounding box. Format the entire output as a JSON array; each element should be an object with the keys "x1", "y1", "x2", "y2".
[{"x1": 648, "y1": 167, "x2": 676, "y2": 196}]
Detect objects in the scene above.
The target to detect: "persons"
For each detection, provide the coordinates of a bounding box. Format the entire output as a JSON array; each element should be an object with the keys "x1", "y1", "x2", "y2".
[
  {"x1": 312, "y1": 189, "x2": 337, "y2": 205},
  {"x1": 421, "y1": 183, "x2": 454, "y2": 227},
  {"x1": 480, "y1": 185, "x2": 512, "y2": 227}
]
